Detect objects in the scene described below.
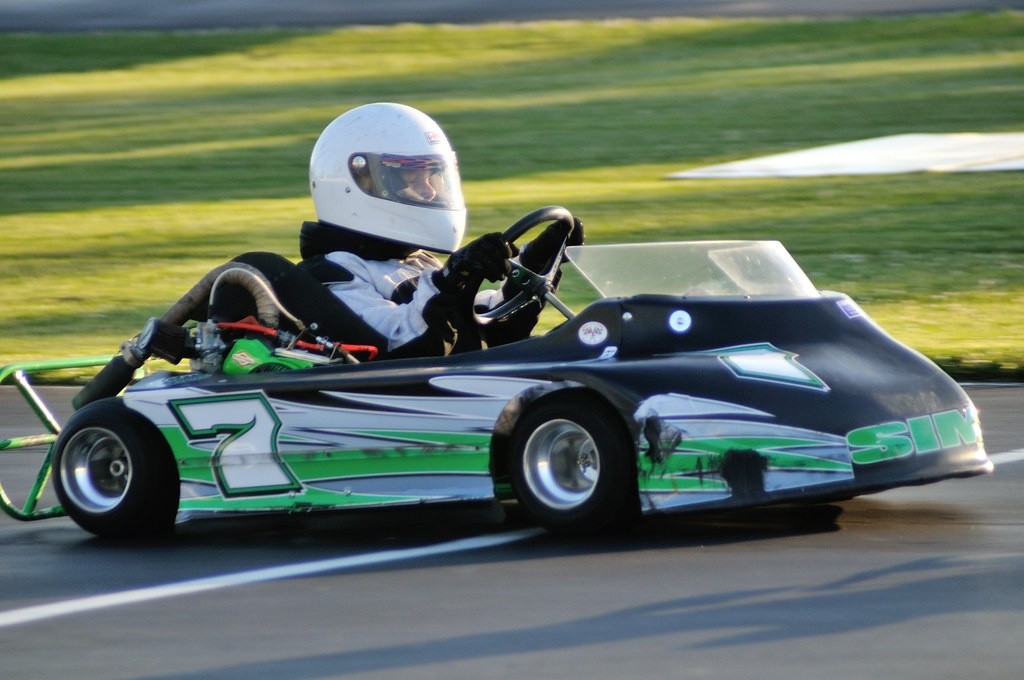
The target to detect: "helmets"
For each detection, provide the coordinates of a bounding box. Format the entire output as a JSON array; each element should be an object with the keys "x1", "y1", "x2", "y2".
[{"x1": 308, "y1": 102, "x2": 467, "y2": 255}]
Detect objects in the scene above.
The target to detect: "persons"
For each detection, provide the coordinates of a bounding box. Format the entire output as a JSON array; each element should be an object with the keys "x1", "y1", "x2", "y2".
[{"x1": 286, "y1": 100, "x2": 586, "y2": 368}]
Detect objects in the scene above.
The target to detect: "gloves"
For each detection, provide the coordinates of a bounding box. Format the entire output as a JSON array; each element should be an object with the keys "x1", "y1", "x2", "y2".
[
  {"x1": 436, "y1": 232, "x2": 519, "y2": 299},
  {"x1": 520, "y1": 217, "x2": 585, "y2": 291}
]
